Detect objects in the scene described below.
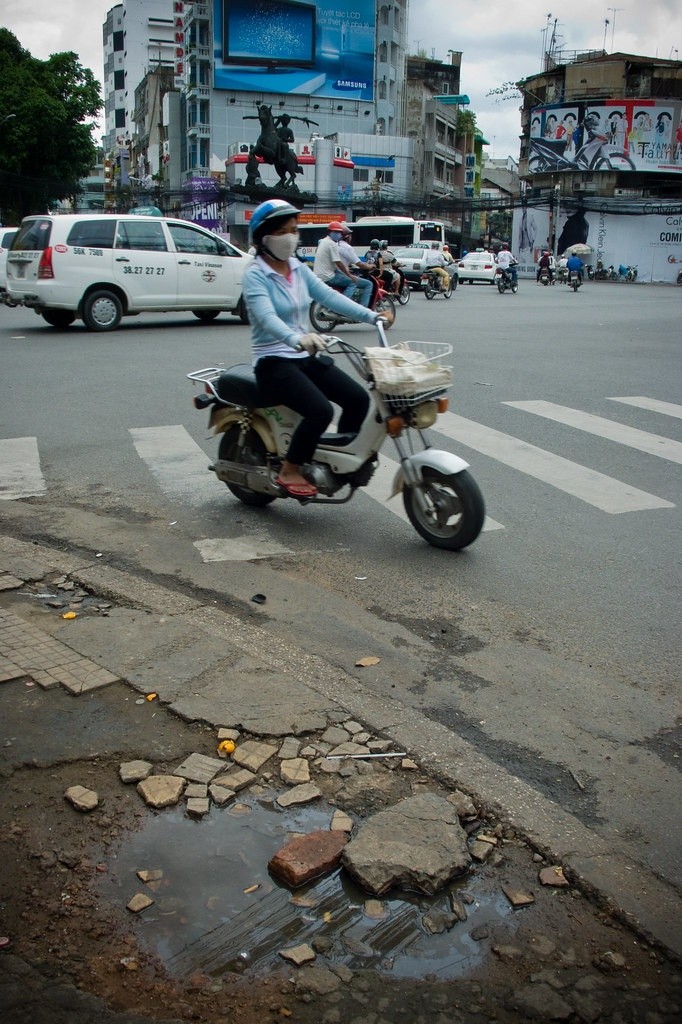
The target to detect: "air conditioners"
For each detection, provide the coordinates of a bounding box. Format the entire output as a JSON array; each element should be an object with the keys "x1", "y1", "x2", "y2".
[{"x1": 572, "y1": 181, "x2": 586, "y2": 192}]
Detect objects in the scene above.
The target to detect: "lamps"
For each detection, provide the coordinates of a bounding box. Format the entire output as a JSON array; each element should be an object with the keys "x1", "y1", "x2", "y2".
[
  {"x1": 580, "y1": 78, "x2": 590, "y2": 94},
  {"x1": 279, "y1": 102, "x2": 284, "y2": 106},
  {"x1": 356, "y1": 110, "x2": 371, "y2": 118},
  {"x1": 338, "y1": 105, "x2": 343, "y2": 110},
  {"x1": 314, "y1": 104, "x2": 319, "y2": 110},
  {"x1": 230, "y1": 98, "x2": 236, "y2": 103},
  {"x1": 256, "y1": 101, "x2": 260, "y2": 105}
]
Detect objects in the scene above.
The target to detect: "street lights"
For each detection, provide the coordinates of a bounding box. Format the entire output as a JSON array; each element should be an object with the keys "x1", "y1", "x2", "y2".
[{"x1": 380, "y1": 154, "x2": 395, "y2": 215}]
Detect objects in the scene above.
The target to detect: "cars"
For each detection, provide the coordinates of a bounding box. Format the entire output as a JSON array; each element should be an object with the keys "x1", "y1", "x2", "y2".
[
  {"x1": 458, "y1": 248, "x2": 497, "y2": 285},
  {"x1": 393, "y1": 244, "x2": 458, "y2": 291}
]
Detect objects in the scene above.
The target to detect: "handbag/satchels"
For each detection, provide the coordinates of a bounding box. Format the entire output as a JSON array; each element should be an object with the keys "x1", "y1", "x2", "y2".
[{"x1": 364, "y1": 342, "x2": 452, "y2": 396}]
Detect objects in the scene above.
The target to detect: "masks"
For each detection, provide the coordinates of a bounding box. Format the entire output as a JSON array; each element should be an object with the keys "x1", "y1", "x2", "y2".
[
  {"x1": 330, "y1": 232, "x2": 342, "y2": 241},
  {"x1": 262, "y1": 233, "x2": 298, "y2": 260}
]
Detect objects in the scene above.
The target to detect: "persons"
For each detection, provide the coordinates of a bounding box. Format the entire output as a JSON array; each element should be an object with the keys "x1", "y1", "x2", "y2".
[
  {"x1": 271, "y1": 114, "x2": 295, "y2": 180},
  {"x1": 426, "y1": 243, "x2": 451, "y2": 292},
  {"x1": 544, "y1": 113, "x2": 672, "y2": 153},
  {"x1": 441, "y1": 245, "x2": 455, "y2": 263},
  {"x1": 313, "y1": 221, "x2": 376, "y2": 307},
  {"x1": 536, "y1": 251, "x2": 584, "y2": 286},
  {"x1": 242, "y1": 199, "x2": 394, "y2": 496},
  {"x1": 498, "y1": 245, "x2": 519, "y2": 284},
  {"x1": 364, "y1": 239, "x2": 403, "y2": 296}
]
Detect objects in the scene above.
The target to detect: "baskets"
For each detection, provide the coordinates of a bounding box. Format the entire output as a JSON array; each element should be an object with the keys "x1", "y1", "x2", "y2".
[{"x1": 361, "y1": 341, "x2": 454, "y2": 413}]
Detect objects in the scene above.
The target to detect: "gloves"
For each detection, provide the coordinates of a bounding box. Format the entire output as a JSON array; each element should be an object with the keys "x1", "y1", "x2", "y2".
[{"x1": 299, "y1": 333, "x2": 327, "y2": 357}]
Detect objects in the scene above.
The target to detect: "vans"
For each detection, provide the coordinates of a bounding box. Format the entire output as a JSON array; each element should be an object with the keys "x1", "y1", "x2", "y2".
[{"x1": 0, "y1": 214, "x2": 251, "y2": 332}]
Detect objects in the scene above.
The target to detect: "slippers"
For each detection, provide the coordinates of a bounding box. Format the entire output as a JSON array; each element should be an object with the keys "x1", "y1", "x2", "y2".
[{"x1": 276, "y1": 478, "x2": 318, "y2": 495}]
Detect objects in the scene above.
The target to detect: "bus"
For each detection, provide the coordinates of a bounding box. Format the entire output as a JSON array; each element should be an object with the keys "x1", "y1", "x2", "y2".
[{"x1": 296, "y1": 215, "x2": 445, "y2": 263}]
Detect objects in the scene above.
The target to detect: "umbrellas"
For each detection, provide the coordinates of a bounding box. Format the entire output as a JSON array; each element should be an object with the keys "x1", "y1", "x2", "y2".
[{"x1": 567, "y1": 243, "x2": 595, "y2": 255}]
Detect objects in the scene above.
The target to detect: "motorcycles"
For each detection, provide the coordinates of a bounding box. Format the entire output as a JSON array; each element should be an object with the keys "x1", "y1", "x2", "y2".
[
  {"x1": 309, "y1": 263, "x2": 411, "y2": 332},
  {"x1": 677, "y1": 260, "x2": 682, "y2": 284},
  {"x1": 495, "y1": 262, "x2": 519, "y2": 294},
  {"x1": 186, "y1": 315, "x2": 486, "y2": 551},
  {"x1": 420, "y1": 262, "x2": 452, "y2": 300},
  {"x1": 537, "y1": 267, "x2": 584, "y2": 292},
  {"x1": 528, "y1": 104, "x2": 636, "y2": 172}
]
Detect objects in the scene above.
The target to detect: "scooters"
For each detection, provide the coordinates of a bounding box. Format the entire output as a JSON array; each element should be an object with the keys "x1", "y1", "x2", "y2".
[{"x1": 584, "y1": 264, "x2": 638, "y2": 282}]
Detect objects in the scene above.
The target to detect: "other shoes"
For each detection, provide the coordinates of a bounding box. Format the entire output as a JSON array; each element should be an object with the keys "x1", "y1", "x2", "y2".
[
  {"x1": 395, "y1": 293, "x2": 400, "y2": 296},
  {"x1": 513, "y1": 282, "x2": 518, "y2": 285}
]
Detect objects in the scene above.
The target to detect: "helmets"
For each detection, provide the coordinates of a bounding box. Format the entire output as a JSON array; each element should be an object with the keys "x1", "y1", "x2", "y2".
[
  {"x1": 572, "y1": 250, "x2": 577, "y2": 254},
  {"x1": 561, "y1": 255, "x2": 565, "y2": 257},
  {"x1": 341, "y1": 226, "x2": 353, "y2": 236},
  {"x1": 544, "y1": 252, "x2": 549, "y2": 256},
  {"x1": 432, "y1": 241, "x2": 439, "y2": 247},
  {"x1": 249, "y1": 199, "x2": 303, "y2": 235},
  {"x1": 379, "y1": 240, "x2": 388, "y2": 248},
  {"x1": 371, "y1": 239, "x2": 378, "y2": 247},
  {"x1": 502, "y1": 242, "x2": 508, "y2": 246},
  {"x1": 328, "y1": 221, "x2": 343, "y2": 231},
  {"x1": 443, "y1": 245, "x2": 449, "y2": 251}
]
